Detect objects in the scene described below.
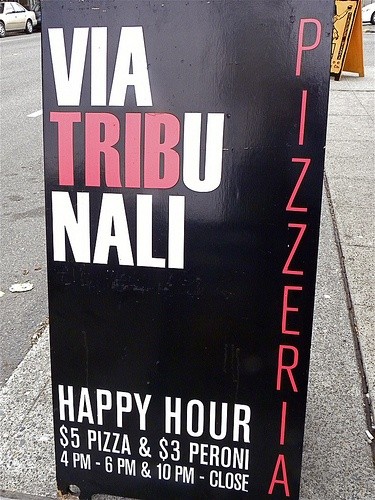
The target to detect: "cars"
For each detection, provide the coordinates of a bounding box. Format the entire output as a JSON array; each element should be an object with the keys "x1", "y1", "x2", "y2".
[{"x1": 0, "y1": 2, "x2": 37, "y2": 37}]
